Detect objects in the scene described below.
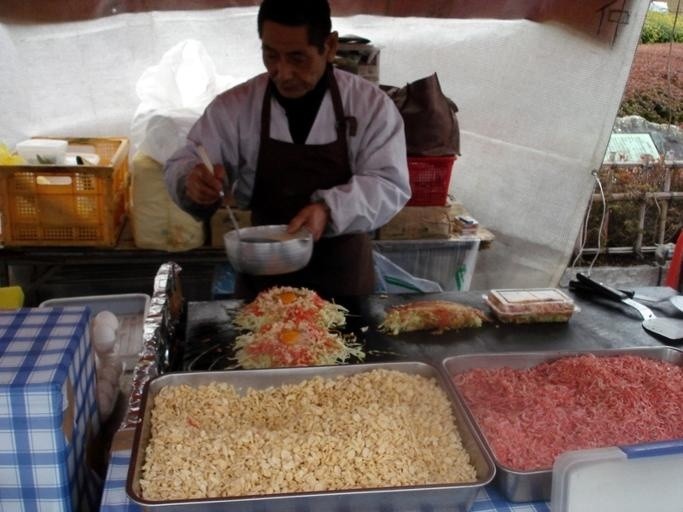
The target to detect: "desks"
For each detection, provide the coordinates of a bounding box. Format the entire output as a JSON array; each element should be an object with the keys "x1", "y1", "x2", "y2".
[{"x1": 2, "y1": 192, "x2": 497, "y2": 291}]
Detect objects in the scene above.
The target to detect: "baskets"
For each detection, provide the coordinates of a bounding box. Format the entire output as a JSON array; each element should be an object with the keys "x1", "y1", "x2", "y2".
[
  {"x1": 0, "y1": 137, "x2": 131, "y2": 249},
  {"x1": 406, "y1": 155, "x2": 457, "y2": 206}
]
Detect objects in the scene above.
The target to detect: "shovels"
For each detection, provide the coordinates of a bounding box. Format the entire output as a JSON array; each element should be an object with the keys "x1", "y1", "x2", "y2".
[{"x1": 569, "y1": 272, "x2": 683, "y2": 340}]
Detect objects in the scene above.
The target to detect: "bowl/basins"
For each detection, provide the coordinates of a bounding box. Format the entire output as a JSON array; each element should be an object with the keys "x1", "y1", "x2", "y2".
[
  {"x1": 16, "y1": 139, "x2": 68, "y2": 167},
  {"x1": 222, "y1": 223, "x2": 313, "y2": 275}
]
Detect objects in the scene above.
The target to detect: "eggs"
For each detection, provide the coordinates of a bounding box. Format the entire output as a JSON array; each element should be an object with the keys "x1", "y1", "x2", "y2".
[
  {"x1": 93, "y1": 310, "x2": 124, "y2": 414},
  {"x1": 280, "y1": 330, "x2": 299, "y2": 345},
  {"x1": 280, "y1": 292, "x2": 296, "y2": 304}
]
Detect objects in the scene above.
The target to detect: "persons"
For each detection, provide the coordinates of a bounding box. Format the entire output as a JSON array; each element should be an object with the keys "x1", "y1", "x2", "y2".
[{"x1": 163, "y1": 0, "x2": 413, "y2": 300}]
